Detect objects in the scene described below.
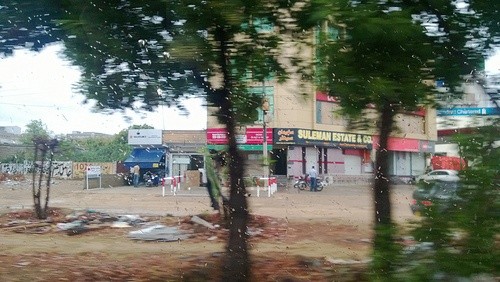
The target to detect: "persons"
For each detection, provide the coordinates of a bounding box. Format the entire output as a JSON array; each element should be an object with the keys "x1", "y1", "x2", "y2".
[
  {"x1": 133, "y1": 165, "x2": 140, "y2": 187},
  {"x1": 310, "y1": 166, "x2": 317, "y2": 192}
]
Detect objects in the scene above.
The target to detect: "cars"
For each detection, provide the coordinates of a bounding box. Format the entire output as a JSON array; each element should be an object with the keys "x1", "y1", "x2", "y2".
[
  {"x1": 410, "y1": 181, "x2": 477, "y2": 217},
  {"x1": 415, "y1": 169, "x2": 460, "y2": 184}
]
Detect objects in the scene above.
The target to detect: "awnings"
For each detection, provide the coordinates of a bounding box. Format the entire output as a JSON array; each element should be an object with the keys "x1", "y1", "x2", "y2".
[{"x1": 124, "y1": 148, "x2": 164, "y2": 169}]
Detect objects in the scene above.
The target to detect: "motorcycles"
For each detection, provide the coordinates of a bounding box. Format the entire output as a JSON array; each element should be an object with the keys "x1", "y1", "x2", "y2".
[{"x1": 294, "y1": 174, "x2": 324, "y2": 192}]
